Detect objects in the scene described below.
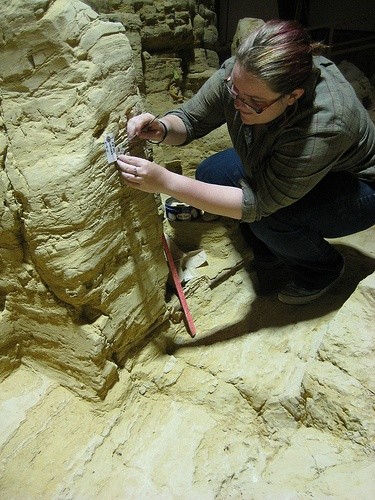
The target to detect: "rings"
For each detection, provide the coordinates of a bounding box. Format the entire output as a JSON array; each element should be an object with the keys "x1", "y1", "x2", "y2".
[{"x1": 133, "y1": 167, "x2": 137, "y2": 175}]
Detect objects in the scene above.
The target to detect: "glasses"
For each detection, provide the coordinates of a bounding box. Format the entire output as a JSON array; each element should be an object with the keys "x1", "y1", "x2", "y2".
[{"x1": 224, "y1": 75, "x2": 288, "y2": 114}]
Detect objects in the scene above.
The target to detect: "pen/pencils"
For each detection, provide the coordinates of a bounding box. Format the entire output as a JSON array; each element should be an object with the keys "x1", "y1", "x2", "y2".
[{"x1": 121, "y1": 113, "x2": 161, "y2": 150}]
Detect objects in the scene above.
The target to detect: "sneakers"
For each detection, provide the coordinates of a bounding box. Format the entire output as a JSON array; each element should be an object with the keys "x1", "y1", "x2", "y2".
[{"x1": 278, "y1": 245, "x2": 346, "y2": 304}]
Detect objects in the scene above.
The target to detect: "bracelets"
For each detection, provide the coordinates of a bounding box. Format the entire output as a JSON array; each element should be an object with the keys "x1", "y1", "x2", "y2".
[{"x1": 147, "y1": 121, "x2": 168, "y2": 145}]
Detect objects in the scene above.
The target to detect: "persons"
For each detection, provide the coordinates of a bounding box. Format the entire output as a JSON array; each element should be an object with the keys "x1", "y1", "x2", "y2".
[{"x1": 115, "y1": 20, "x2": 375, "y2": 304}]
